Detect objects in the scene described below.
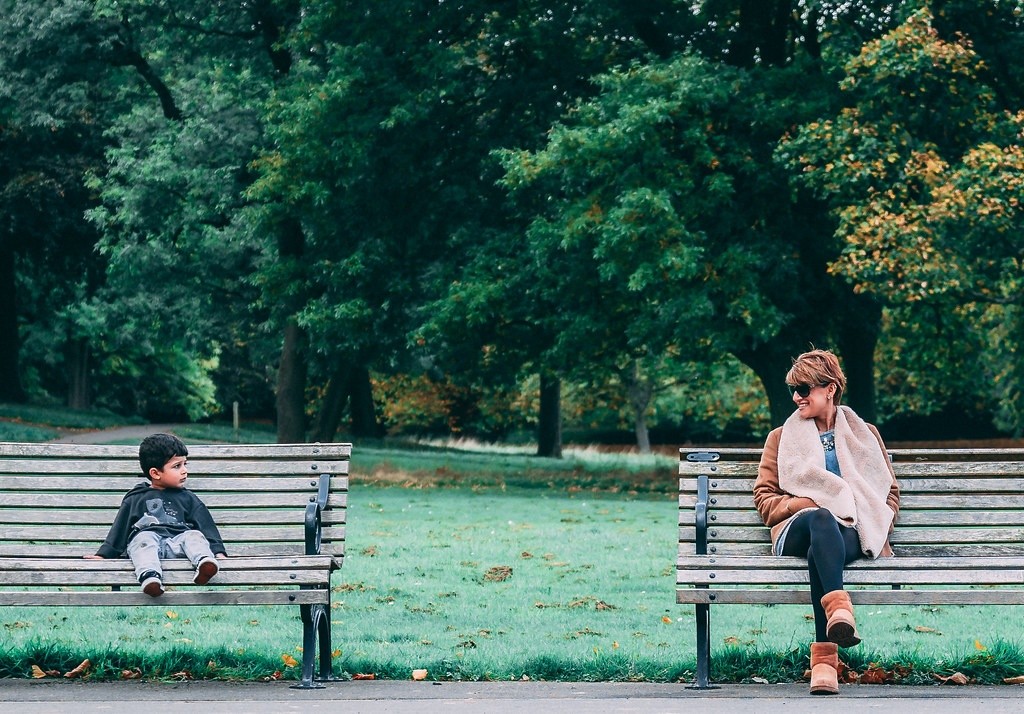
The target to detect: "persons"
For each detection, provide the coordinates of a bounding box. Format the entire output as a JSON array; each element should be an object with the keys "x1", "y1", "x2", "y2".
[
  {"x1": 82, "y1": 432, "x2": 229, "y2": 599},
  {"x1": 752, "y1": 347, "x2": 900, "y2": 694}
]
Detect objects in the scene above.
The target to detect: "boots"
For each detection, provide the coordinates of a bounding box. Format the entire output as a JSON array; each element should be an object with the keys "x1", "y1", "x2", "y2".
[
  {"x1": 821, "y1": 588, "x2": 861, "y2": 648},
  {"x1": 809, "y1": 641, "x2": 839, "y2": 695}
]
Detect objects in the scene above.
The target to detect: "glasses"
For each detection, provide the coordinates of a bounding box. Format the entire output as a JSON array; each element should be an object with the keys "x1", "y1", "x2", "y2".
[{"x1": 788, "y1": 382, "x2": 830, "y2": 398}]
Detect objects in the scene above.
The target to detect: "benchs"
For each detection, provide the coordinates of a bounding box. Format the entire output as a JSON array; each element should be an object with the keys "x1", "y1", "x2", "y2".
[
  {"x1": 677, "y1": 449, "x2": 1024, "y2": 689},
  {"x1": 0, "y1": 442, "x2": 352, "y2": 689}
]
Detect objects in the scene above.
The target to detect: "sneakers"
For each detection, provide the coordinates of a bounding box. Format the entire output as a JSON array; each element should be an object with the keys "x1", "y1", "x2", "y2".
[
  {"x1": 193, "y1": 556, "x2": 219, "y2": 585},
  {"x1": 139, "y1": 570, "x2": 165, "y2": 597}
]
